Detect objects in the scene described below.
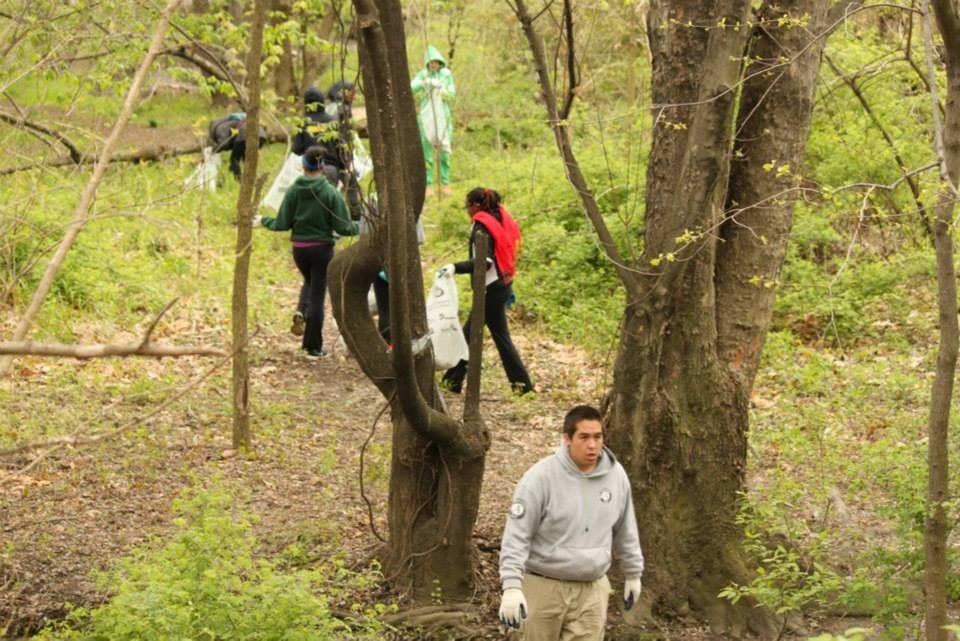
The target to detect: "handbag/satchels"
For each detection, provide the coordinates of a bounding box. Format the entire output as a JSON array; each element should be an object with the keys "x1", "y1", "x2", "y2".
[
  {"x1": 421, "y1": 78, "x2": 451, "y2": 153},
  {"x1": 425, "y1": 271, "x2": 470, "y2": 371},
  {"x1": 185, "y1": 145, "x2": 223, "y2": 192}
]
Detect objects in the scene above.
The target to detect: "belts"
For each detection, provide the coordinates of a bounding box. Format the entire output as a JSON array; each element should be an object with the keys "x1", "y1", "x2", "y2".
[{"x1": 528, "y1": 571, "x2": 594, "y2": 584}]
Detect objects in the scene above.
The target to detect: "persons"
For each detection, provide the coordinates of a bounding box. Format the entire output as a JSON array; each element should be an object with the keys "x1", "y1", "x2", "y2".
[
  {"x1": 210, "y1": 113, "x2": 266, "y2": 181},
  {"x1": 254, "y1": 81, "x2": 424, "y2": 361},
  {"x1": 410, "y1": 45, "x2": 456, "y2": 198},
  {"x1": 441, "y1": 187, "x2": 538, "y2": 397},
  {"x1": 498, "y1": 406, "x2": 644, "y2": 641}
]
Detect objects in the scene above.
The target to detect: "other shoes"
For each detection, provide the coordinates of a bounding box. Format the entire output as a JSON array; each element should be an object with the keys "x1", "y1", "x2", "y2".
[
  {"x1": 425, "y1": 186, "x2": 433, "y2": 195},
  {"x1": 443, "y1": 185, "x2": 451, "y2": 193},
  {"x1": 306, "y1": 349, "x2": 323, "y2": 359},
  {"x1": 291, "y1": 312, "x2": 303, "y2": 336}
]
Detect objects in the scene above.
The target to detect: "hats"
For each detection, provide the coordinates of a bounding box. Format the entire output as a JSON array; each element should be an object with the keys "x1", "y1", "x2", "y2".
[{"x1": 303, "y1": 146, "x2": 328, "y2": 170}]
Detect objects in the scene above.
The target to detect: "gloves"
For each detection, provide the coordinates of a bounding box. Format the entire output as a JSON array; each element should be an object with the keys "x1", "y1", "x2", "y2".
[
  {"x1": 440, "y1": 264, "x2": 456, "y2": 279},
  {"x1": 252, "y1": 216, "x2": 262, "y2": 227},
  {"x1": 624, "y1": 576, "x2": 641, "y2": 610},
  {"x1": 499, "y1": 588, "x2": 528, "y2": 628}
]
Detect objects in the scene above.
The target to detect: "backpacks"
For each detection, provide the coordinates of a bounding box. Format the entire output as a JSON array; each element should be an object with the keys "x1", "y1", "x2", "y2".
[{"x1": 214, "y1": 112, "x2": 248, "y2": 140}]
